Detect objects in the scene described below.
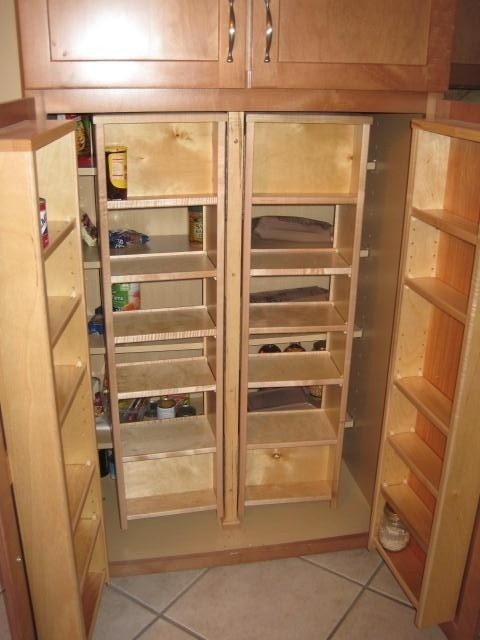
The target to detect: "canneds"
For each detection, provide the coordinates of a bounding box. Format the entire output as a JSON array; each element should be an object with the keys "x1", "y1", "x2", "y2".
[
  {"x1": 188, "y1": 205, "x2": 202, "y2": 250},
  {"x1": 106, "y1": 146, "x2": 128, "y2": 201},
  {"x1": 112, "y1": 282, "x2": 141, "y2": 312},
  {"x1": 37, "y1": 197, "x2": 49, "y2": 248}
]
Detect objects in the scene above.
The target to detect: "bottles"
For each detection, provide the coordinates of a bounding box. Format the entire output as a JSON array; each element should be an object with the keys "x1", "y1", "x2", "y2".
[
  {"x1": 256, "y1": 341, "x2": 328, "y2": 406},
  {"x1": 177, "y1": 399, "x2": 197, "y2": 416},
  {"x1": 379, "y1": 503, "x2": 410, "y2": 552}
]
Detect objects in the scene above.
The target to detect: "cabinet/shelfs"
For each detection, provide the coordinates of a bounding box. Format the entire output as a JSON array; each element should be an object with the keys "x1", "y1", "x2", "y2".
[
  {"x1": 1, "y1": 119, "x2": 108, "y2": 640},
  {"x1": 365, "y1": 111, "x2": 480, "y2": 629},
  {"x1": 238, "y1": 114, "x2": 374, "y2": 522},
  {"x1": 90, "y1": 115, "x2": 225, "y2": 533},
  {"x1": 15, "y1": 0, "x2": 458, "y2": 93}
]
